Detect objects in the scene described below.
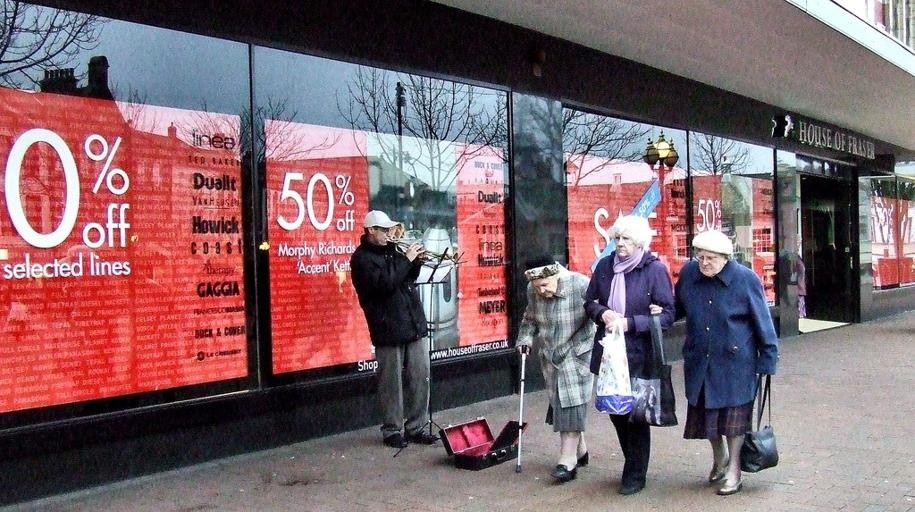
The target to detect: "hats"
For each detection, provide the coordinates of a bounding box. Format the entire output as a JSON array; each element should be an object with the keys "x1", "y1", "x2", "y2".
[
  {"x1": 692, "y1": 230, "x2": 734, "y2": 256},
  {"x1": 524, "y1": 253, "x2": 559, "y2": 281},
  {"x1": 362, "y1": 210, "x2": 399, "y2": 228}
]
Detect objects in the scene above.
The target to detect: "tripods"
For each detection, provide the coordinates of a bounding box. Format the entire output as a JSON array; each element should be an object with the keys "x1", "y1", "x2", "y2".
[{"x1": 391, "y1": 285, "x2": 442, "y2": 459}]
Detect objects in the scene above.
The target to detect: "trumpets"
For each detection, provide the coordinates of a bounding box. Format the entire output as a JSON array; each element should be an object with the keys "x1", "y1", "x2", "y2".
[{"x1": 384, "y1": 238, "x2": 458, "y2": 265}]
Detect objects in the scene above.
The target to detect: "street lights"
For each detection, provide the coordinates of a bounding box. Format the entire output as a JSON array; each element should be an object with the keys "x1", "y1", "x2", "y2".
[{"x1": 642, "y1": 129, "x2": 679, "y2": 255}]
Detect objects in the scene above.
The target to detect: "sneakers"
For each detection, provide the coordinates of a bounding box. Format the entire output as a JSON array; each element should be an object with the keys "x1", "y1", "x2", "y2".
[
  {"x1": 383, "y1": 430, "x2": 436, "y2": 447},
  {"x1": 550, "y1": 451, "x2": 588, "y2": 481}
]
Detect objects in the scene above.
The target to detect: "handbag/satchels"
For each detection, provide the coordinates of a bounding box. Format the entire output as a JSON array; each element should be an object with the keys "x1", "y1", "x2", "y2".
[
  {"x1": 739, "y1": 426, "x2": 778, "y2": 472},
  {"x1": 594, "y1": 316, "x2": 634, "y2": 415},
  {"x1": 626, "y1": 363, "x2": 679, "y2": 426}
]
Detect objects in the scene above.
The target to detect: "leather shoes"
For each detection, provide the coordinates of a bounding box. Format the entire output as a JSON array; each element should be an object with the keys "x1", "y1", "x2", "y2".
[
  {"x1": 709, "y1": 459, "x2": 743, "y2": 495},
  {"x1": 618, "y1": 480, "x2": 646, "y2": 494}
]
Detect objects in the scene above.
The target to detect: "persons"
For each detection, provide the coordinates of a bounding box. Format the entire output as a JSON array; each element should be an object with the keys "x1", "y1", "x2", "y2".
[
  {"x1": 648, "y1": 230, "x2": 782, "y2": 496},
  {"x1": 600, "y1": 213, "x2": 677, "y2": 497},
  {"x1": 513, "y1": 254, "x2": 606, "y2": 481},
  {"x1": 795, "y1": 244, "x2": 808, "y2": 336},
  {"x1": 349, "y1": 210, "x2": 439, "y2": 448}
]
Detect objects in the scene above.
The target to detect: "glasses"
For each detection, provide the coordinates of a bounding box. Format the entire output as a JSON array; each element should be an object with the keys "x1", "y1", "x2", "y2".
[{"x1": 696, "y1": 253, "x2": 726, "y2": 261}]
